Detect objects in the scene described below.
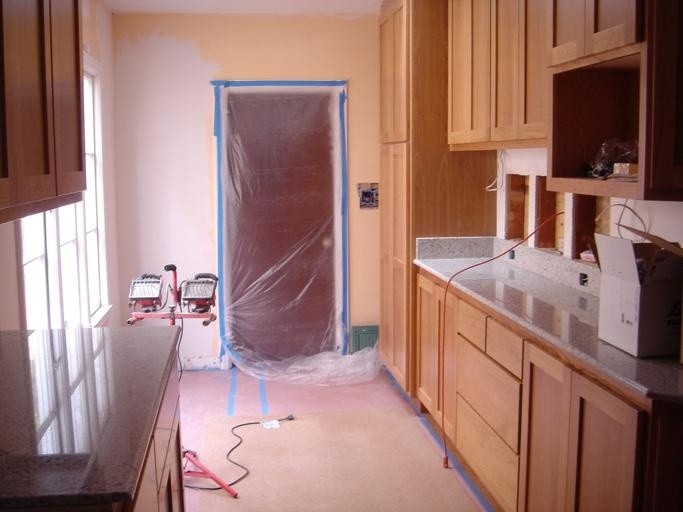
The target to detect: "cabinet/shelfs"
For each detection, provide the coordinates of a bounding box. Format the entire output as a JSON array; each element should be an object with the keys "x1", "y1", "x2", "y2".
[
  {"x1": 0, "y1": 0, "x2": 86, "y2": 225},
  {"x1": 545, "y1": 2, "x2": 682, "y2": 200},
  {"x1": 525, "y1": 329, "x2": 683, "y2": 512},
  {"x1": 449, "y1": 0, "x2": 550, "y2": 149},
  {"x1": 113, "y1": 350, "x2": 186, "y2": 512},
  {"x1": 459, "y1": 287, "x2": 525, "y2": 512},
  {"x1": 375, "y1": 1, "x2": 500, "y2": 416},
  {"x1": 410, "y1": 261, "x2": 459, "y2": 451}
]
